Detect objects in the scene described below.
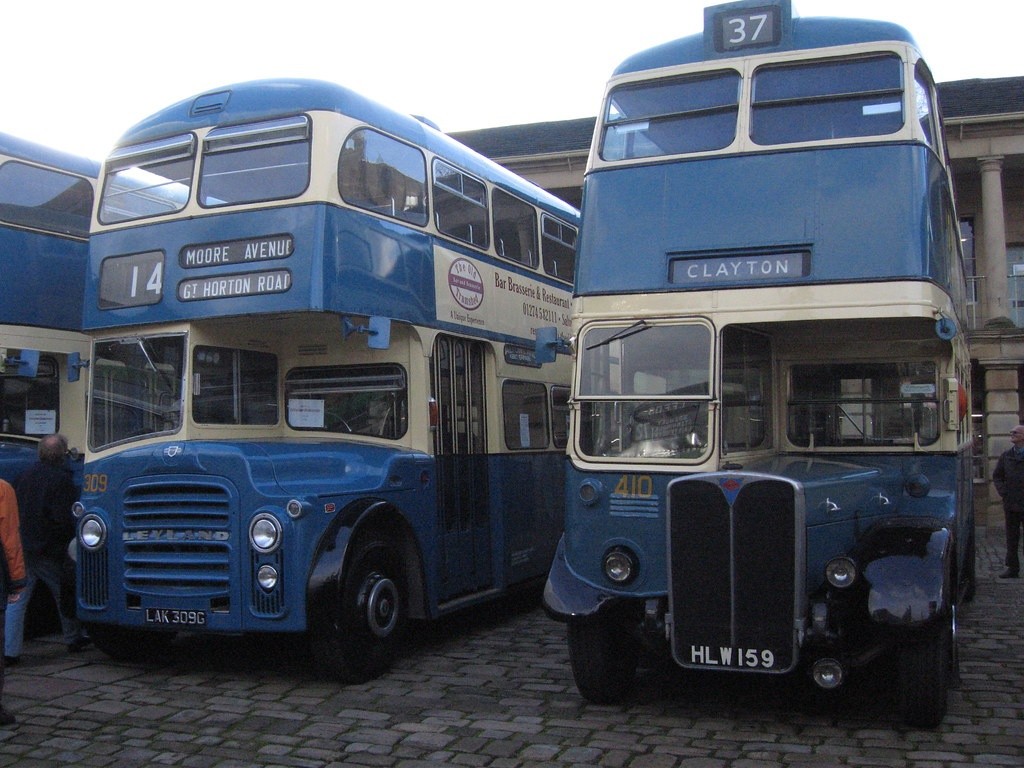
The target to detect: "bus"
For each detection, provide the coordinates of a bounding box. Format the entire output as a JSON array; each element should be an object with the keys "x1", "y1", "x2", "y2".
[
  {"x1": 535, "y1": 0, "x2": 975, "y2": 729},
  {"x1": 66, "y1": 77, "x2": 620, "y2": 684},
  {"x1": 0, "y1": 132, "x2": 229, "y2": 635}
]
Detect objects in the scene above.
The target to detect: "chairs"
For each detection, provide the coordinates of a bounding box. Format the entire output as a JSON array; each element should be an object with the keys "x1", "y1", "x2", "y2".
[{"x1": 358, "y1": 196, "x2": 558, "y2": 277}]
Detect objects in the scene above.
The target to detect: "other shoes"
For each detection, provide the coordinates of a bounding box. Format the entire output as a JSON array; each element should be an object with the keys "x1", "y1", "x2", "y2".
[
  {"x1": 4, "y1": 656, "x2": 20, "y2": 668},
  {"x1": 67, "y1": 636, "x2": 94, "y2": 653},
  {"x1": 999, "y1": 569, "x2": 1018, "y2": 579},
  {"x1": 0, "y1": 713, "x2": 15, "y2": 726}
]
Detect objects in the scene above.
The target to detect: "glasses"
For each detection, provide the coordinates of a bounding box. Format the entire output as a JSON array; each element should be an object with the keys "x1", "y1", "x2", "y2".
[{"x1": 1010, "y1": 430, "x2": 1024, "y2": 435}]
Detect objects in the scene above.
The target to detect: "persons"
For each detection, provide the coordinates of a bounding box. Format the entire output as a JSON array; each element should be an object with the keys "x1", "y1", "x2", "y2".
[
  {"x1": 4, "y1": 433, "x2": 93, "y2": 664},
  {"x1": 0, "y1": 478, "x2": 29, "y2": 727},
  {"x1": 992, "y1": 424, "x2": 1024, "y2": 579}
]
